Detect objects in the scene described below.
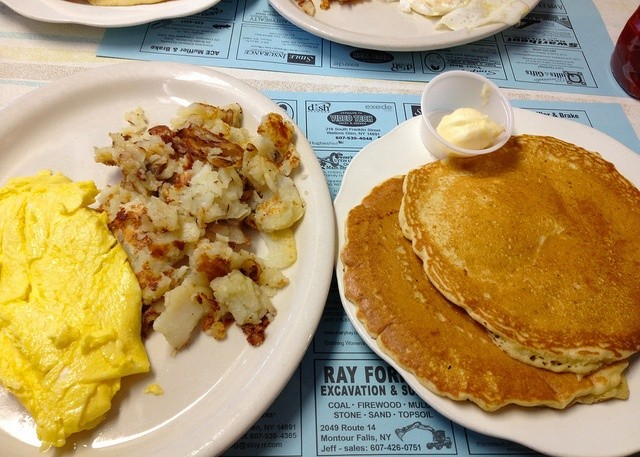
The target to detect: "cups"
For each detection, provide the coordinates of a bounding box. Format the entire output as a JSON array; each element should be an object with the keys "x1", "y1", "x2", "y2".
[{"x1": 418, "y1": 69, "x2": 515, "y2": 158}]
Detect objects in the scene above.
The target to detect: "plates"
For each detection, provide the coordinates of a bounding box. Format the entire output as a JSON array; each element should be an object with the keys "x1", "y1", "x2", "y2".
[
  {"x1": 334, "y1": 107, "x2": 638, "y2": 457},
  {"x1": 268, "y1": 0, "x2": 541, "y2": 52},
  {"x1": 0, "y1": 0, "x2": 219, "y2": 30},
  {"x1": 0, "y1": 60, "x2": 334, "y2": 457}
]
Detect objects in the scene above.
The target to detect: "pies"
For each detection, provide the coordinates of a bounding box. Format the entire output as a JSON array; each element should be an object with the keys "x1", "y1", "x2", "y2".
[
  {"x1": 340, "y1": 173, "x2": 630, "y2": 412},
  {"x1": 401, "y1": 135, "x2": 640, "y2": 374}
]
